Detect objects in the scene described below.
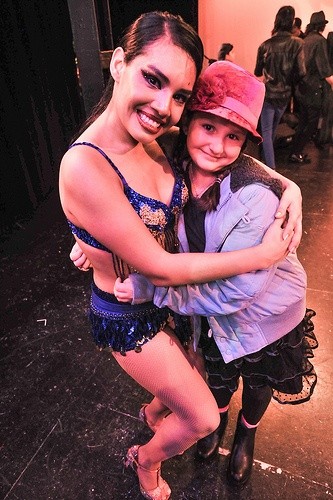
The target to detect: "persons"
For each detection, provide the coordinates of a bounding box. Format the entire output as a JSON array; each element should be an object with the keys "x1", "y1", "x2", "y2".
[
  {"x1": 57, "y1": 9, "x2": 303, "y2": 500},
  {"x1": 70, "y1": 59, "x2": 319, "y2": 488},
  {"x1": 208, "y1": 6, "x2": 333, "y2": 174}
]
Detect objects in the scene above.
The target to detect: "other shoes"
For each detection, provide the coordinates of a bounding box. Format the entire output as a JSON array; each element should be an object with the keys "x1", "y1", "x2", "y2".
[
  {"x1": 321, "y1": 143, "x2": 330, "y2": 157},
  {"x1": 287, "y1": 154, "x2": 311, "y2": 164},
  {"x1": 297, "y1": 153, "x2": 309, "y2": 158}
]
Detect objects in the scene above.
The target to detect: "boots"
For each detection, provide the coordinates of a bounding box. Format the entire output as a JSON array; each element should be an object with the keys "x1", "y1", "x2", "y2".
[
  {"x1": 195, "y1": 411, "x2": 229, "y2": 462},
  {"x1": 227, "y1": 410, "x2": 257, "y2": 485}
]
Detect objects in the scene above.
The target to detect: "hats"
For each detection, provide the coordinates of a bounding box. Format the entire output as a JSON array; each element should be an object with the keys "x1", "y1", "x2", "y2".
[
  {"x1": 187, "y1": 60, "x2": 266, "y2": 145},
  {"x1": 307, "y1": 10, "x2": 328, "y2": 27}
]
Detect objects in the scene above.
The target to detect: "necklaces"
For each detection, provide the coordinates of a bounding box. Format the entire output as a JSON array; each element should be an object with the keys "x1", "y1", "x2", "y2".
[{"x1": 190, "y1": 167, "x2": 216, "y2": 199}]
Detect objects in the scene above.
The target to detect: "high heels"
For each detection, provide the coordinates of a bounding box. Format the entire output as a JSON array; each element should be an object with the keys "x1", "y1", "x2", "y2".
[
  {"x1": 123, "y1": 443, "x2": 172, "y2": 500},
  {"x1": 138, "y1": 404, "x2": 185, "y2": 455}
]
